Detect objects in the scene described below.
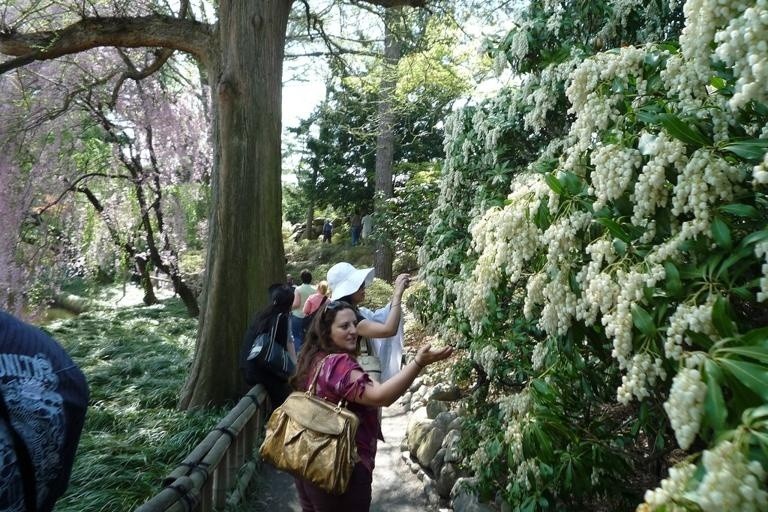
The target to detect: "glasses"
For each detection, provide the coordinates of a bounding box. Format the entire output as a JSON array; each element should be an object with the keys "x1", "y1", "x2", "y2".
[{"x1": 327, "y1": 300, "x2": 353, "y2": 310}]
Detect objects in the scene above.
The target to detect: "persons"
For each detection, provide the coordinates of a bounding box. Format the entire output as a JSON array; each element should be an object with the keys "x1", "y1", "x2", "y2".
[
  {"x1": 350, "y1": 211, "x2": 360, "y2": 245},
  {"x1": 361, "y1": 210, "x2": 374, "y2": 246},
  {"x1": 0, "y1": 307, "x2": 93, "y2": 512},
  {"x1": 326, "y1": 262, "x2": 409, "y2": 383},
  {"x1": 293, "y1": 269, "x2": 315, "y2": 353},
  {"x1": 304, "y1": 279, "x2": 329, "y2": 325},
  {"x1": 242, "y1": 284, "x2": 299, "y2": 411},
  {"x1": 288, "y1": 299, "x2": 455, "y2": 512},
  {"x1": 322, "y1": 218, "x2": 332, "y2": 244},
  {"x1": 285, "y1": 274, "x2": 299, "y2": 309}
]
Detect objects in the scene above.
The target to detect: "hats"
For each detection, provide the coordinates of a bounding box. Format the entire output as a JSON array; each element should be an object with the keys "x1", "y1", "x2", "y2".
[{"x1": 326, "y1": 262, "x2": 375, "y2": 300}]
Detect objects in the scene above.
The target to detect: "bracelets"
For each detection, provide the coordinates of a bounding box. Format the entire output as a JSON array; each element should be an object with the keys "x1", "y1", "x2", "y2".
[
  {"x1": 388, "y1": 300, "x2": 403, "y2": 309},
  {"x1": 413, "y1": 358, "x2": 421, "y2": 368}
]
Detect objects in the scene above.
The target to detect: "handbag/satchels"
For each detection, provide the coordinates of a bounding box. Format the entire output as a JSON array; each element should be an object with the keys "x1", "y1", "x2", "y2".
[
  {"x1": 302, "y1": 313, "x2": 312, "y2": 325},
  {"x1": 259, "y1": 391, "x2": 362, "y2": 494},
  {"x1": 248, "y1": 333, "x2": 294, "y2": 383},
  {"x1": 354, "y1": 355, "x2": 380, "y2": 383}
]
